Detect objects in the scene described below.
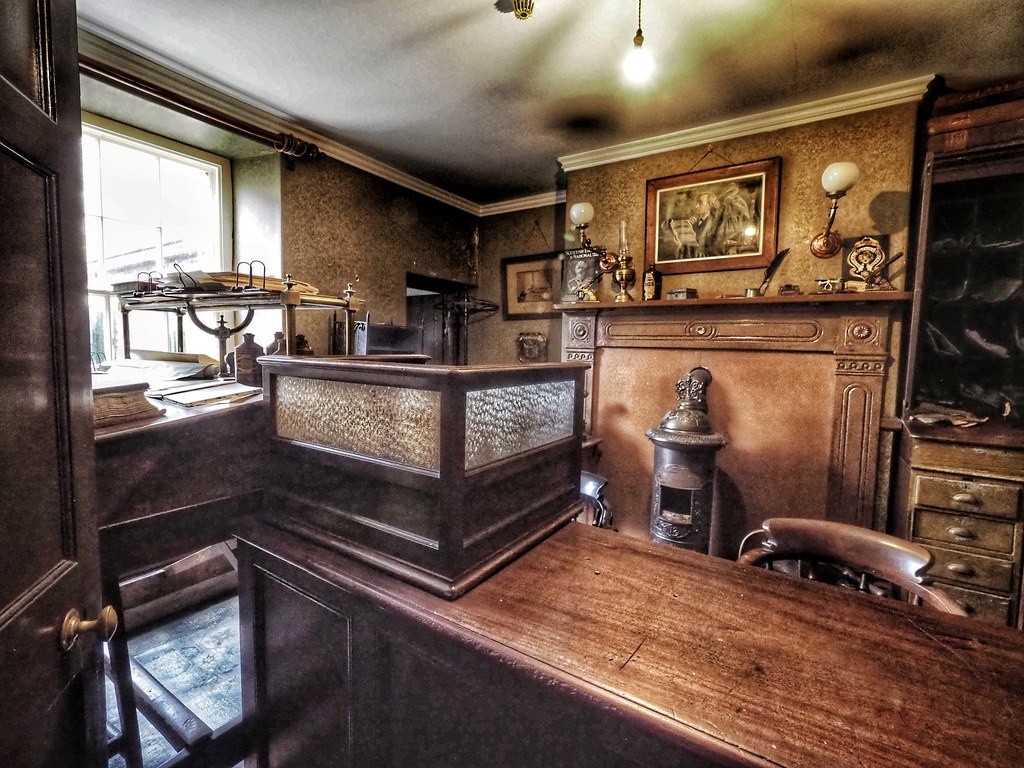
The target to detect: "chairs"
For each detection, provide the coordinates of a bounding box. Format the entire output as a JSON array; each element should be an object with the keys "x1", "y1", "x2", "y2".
[{"x1": 734, "y1": 517, "x2": 973, "y2": 618}]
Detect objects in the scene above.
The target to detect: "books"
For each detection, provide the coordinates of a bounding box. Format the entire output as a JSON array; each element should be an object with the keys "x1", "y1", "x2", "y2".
[{"x1": 93, "y1": 269, "x2": 319, "y2": 429}]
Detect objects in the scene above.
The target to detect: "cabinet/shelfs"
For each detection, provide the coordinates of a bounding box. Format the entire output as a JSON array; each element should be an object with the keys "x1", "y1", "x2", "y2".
[
  {"x1": 892, "y1": 431, "x2": 1024, "y2": 632},
  {"x1": 117, "y1": 274, "x2": 357, "y2": 382},
  {"x1": 255, "y1": 356, "x2": 592, "y2": 602}
]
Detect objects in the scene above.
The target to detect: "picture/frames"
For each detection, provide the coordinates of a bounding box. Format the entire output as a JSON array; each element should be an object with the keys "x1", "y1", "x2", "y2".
[
  {"x1": 645, "y1": 156, "x2": 784, "y2": 276},
  {"x1": 501, "y1": 251, "x2": 564, "y2": 320}
]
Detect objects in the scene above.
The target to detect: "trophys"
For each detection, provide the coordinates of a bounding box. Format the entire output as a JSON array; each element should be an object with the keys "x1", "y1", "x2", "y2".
[{"x1": 612, "y1": 220, "x2": 635, "y2": 303}]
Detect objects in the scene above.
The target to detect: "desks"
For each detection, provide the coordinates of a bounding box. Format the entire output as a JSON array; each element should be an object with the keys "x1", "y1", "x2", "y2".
[
  {"x1": 95, "y1": 385, "x2": 264, "y2": 767},
  {"x1": 232, "y1": 507, "x2": 1023, "y2": 768}
]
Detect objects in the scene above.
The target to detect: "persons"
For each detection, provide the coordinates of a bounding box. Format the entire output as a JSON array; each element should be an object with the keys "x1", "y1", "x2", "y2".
[{"x1": 673, "y1": 192, "x2": 720, "y2": 260}]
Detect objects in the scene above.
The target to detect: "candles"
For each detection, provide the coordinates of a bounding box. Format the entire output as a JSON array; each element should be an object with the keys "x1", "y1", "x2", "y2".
[{"x1": 620, "y1": 221, "x2": 628, "y2": 250}]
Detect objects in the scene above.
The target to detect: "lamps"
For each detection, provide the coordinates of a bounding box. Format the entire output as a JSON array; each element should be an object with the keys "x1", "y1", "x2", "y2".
[
  {"x1": 809, "y1": 161, "x2": 860, "y2": 260},
  {"x1": 569, "y1": 202, "x2": 619, "y2": 273}
]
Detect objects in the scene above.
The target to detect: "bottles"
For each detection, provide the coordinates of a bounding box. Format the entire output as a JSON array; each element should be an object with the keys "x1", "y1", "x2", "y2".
[
  {"x1": 296, "y1": 334, "x2": 314, "y2": 355},
  {"x1": 272, "y1": 340, "x2": 286, "y2": 355},
  {"x1": 234, "y1": 333, "x2": 263, "y2": 387},
  {"x1": 641, "y1": 262, "x2": 662, "y2": 301},
  {"x1": 266, "y1": 332, "x2": 284, "y2": 355}
]
